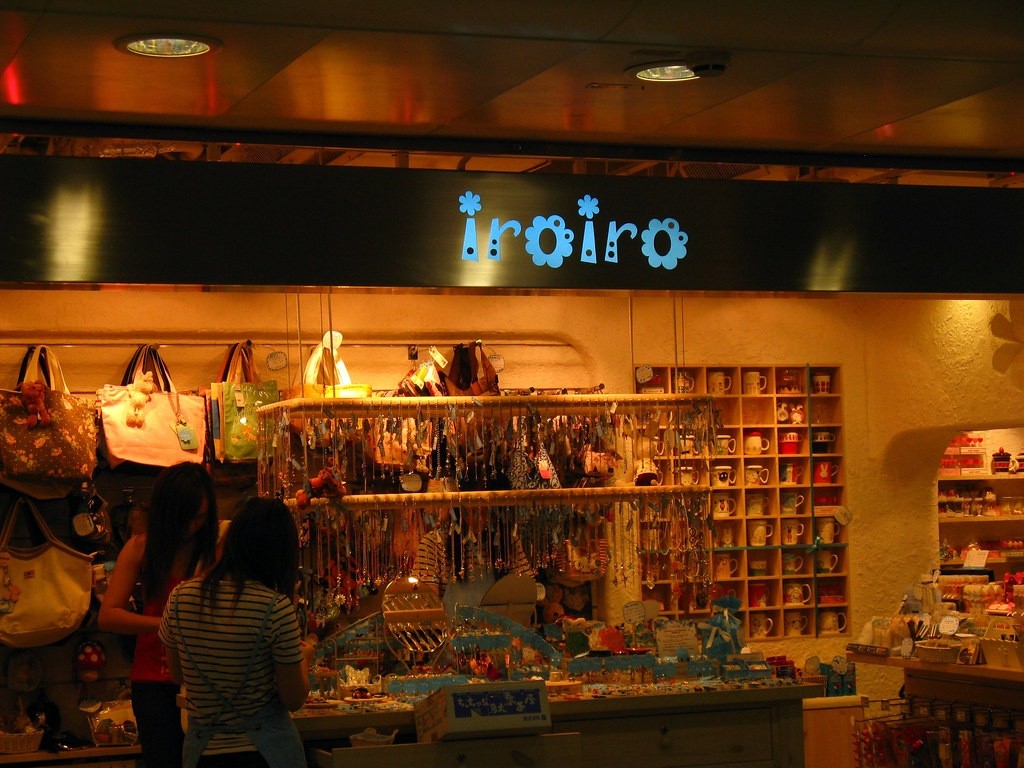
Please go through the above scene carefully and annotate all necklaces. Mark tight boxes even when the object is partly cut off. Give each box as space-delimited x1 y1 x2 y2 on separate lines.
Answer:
258 401 717 631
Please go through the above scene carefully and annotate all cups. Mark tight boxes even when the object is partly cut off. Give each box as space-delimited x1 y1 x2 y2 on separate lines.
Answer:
635 371 848 637
350 727 394 747
1012 453 1024 474
992 447 1017 475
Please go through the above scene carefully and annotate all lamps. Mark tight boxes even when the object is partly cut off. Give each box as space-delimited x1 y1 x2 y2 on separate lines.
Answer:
113 33 225 59
623 60 700 82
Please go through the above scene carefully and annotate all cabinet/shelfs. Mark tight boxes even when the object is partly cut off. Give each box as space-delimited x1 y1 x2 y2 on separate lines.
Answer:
631 362 851 641
0 743 142 768
939 471 1024 573
802 696 869 768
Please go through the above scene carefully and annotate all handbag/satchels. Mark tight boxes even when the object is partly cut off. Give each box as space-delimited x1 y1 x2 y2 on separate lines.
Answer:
96 344 206 470
198 342 283 464
366 343 503 470
508 415 562 490
546 512 608 588
0 496 94 648
0 345 97 500
285 341 373 447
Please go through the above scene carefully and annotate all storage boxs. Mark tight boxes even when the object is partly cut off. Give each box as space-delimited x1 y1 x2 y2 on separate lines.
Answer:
938 447 992 475
415 679 552 744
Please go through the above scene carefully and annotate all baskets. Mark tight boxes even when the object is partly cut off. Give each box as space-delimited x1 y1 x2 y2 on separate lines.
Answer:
0 728 44 754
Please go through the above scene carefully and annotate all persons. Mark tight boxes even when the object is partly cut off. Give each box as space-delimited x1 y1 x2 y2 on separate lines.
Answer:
411 464 536 598
98 462 219 768
159 498 310 768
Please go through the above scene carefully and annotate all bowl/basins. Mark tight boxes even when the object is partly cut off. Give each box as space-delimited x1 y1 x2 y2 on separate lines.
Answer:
915 639 962 664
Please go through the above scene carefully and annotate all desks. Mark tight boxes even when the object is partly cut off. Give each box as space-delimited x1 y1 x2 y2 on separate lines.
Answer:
844 652 1024 712
179 680 822 768
